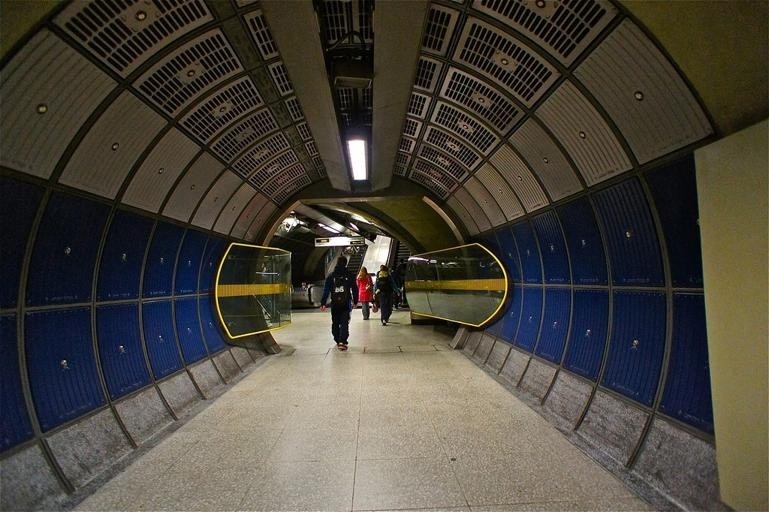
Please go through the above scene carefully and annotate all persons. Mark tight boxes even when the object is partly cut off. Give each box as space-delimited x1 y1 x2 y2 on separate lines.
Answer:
320 256 359 349
356 259 407 326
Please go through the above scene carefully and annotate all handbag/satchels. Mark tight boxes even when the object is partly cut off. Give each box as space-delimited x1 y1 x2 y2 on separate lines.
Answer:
366 283 373 293
372 302 378 312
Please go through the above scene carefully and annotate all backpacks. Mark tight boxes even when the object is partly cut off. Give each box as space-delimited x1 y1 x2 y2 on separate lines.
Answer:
331 272 351 305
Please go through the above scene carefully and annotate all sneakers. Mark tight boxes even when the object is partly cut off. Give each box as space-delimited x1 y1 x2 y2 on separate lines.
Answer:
382 320 386 325
338 342 347 351
